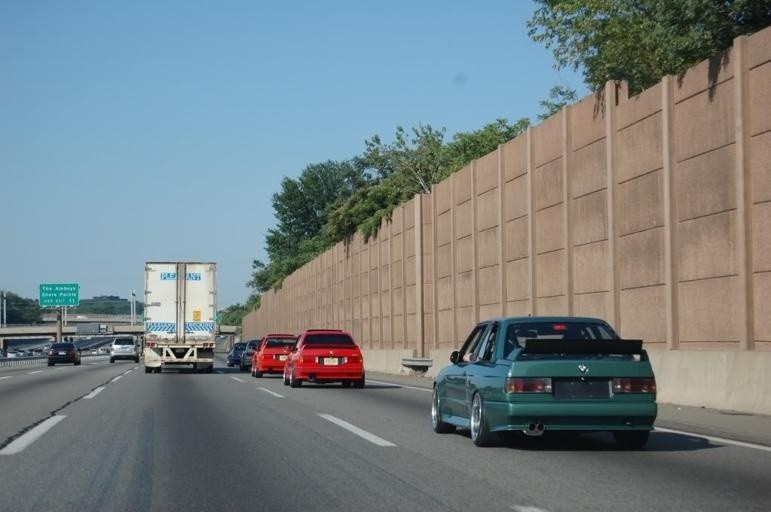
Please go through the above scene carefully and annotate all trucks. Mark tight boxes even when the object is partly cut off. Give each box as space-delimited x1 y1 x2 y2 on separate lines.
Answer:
144 262 216 374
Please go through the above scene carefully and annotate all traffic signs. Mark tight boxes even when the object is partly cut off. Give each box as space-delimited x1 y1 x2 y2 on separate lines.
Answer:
40 284 79 307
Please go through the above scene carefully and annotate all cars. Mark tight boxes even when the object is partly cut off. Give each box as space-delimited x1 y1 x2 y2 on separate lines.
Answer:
6 345 49 358
228 330 365 389
431 316 658 449
48 343 82 366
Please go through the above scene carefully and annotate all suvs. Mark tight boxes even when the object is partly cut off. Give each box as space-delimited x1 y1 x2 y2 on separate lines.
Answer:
109 336 140 363
